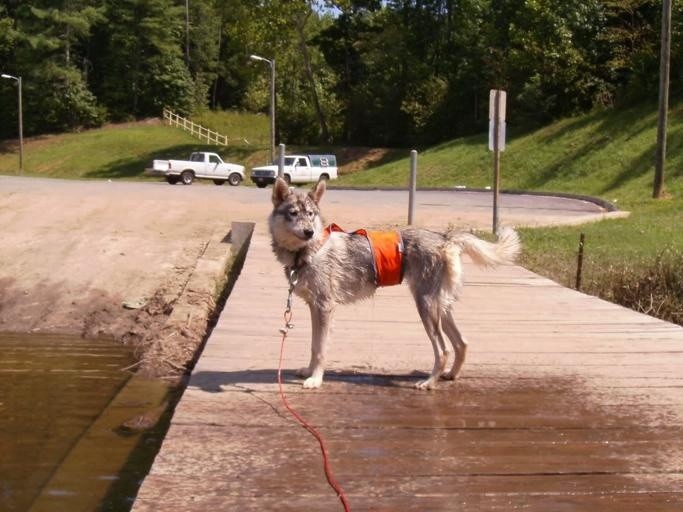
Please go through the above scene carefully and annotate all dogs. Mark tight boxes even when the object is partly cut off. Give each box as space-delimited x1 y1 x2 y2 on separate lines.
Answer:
266 174 522 393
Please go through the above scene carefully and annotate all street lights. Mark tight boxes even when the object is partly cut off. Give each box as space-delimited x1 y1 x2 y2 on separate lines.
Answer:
249 53 275 163
1 74 22 166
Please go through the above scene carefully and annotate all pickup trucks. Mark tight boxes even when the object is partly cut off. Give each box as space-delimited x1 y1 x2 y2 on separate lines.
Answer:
154 152 246 185
250 154 338 188
